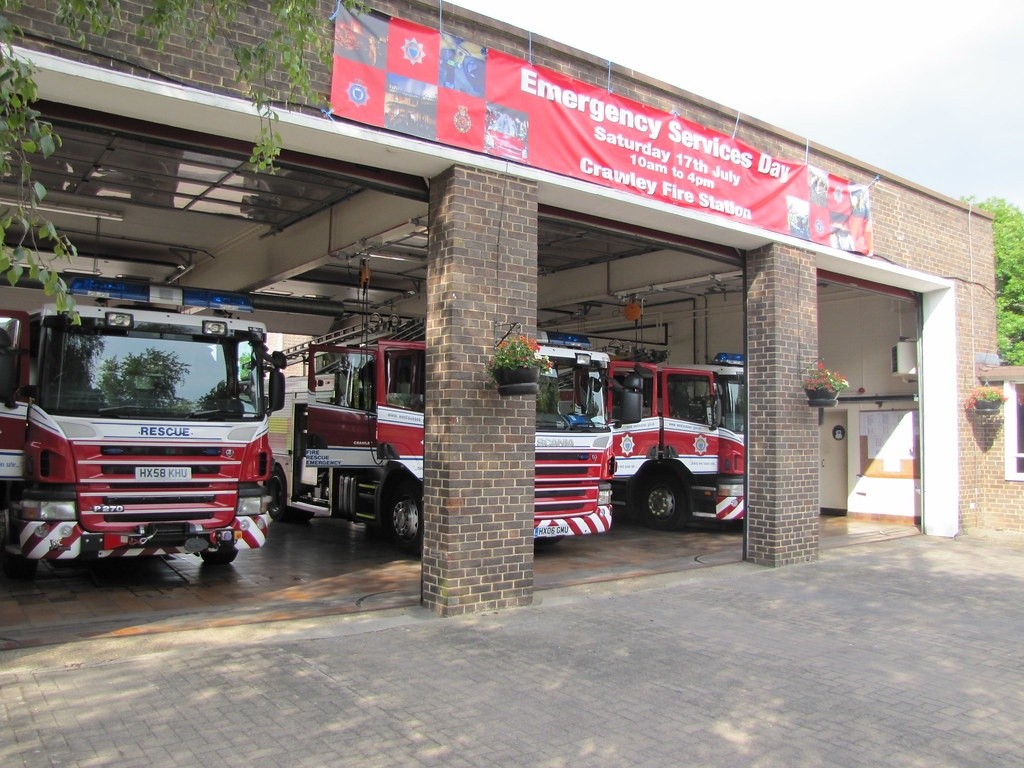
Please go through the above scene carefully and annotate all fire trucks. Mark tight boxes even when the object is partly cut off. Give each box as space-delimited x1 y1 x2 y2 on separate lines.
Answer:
598 338 746 522
261 312 644 558
1 275 288 576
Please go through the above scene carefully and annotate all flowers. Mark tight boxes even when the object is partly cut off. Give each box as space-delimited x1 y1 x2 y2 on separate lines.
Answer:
804 363 850 393
965 386 1008 408
487 334 551 373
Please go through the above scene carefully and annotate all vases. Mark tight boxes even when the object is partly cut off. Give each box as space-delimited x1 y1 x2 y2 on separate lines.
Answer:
806 389 839 408
497 366 539 395
975 400 1000 414
